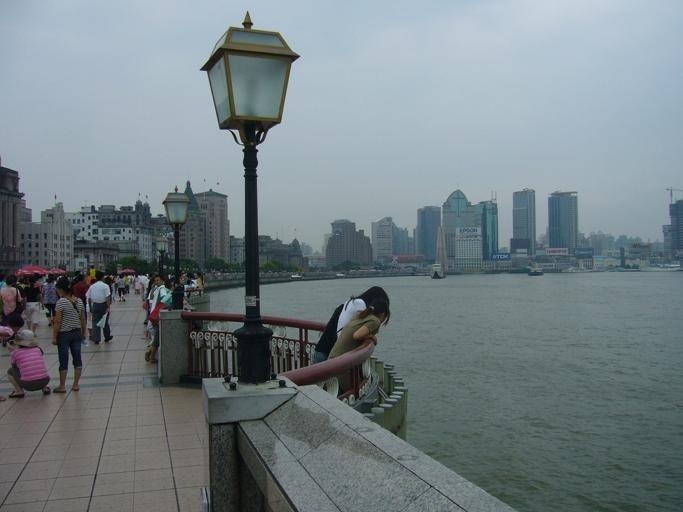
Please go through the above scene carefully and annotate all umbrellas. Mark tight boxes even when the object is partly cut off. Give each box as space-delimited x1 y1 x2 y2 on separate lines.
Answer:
13 263 64 277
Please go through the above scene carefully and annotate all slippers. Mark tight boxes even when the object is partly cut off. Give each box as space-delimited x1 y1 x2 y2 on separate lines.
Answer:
72 387 79 391
52 387 67 393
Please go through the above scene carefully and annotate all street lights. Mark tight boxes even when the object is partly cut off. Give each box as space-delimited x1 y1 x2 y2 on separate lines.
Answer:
157 187 192 310
201 11 299 380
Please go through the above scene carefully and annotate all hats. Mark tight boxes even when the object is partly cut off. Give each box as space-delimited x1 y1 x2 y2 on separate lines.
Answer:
14 329 39 347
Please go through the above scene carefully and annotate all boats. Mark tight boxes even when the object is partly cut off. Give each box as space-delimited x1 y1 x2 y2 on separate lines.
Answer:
430 263 445 278
526 262 543 275
560 266 588 273
610 267 640 273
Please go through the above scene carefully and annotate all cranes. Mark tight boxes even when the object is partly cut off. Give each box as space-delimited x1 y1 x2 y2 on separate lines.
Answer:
662 184 683 204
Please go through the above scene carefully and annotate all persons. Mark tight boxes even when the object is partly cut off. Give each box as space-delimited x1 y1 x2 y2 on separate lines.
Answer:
7 330 51 398
51 276 87 393
0 265 205 363
310 286 389 396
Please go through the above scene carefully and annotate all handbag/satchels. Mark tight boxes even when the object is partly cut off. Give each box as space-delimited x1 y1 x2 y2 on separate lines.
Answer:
15 288 27 314
146 302 168 321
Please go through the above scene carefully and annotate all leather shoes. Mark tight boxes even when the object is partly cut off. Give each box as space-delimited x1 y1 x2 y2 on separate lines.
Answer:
8 390 25 398
94 336 113 345
42 386 51 395
